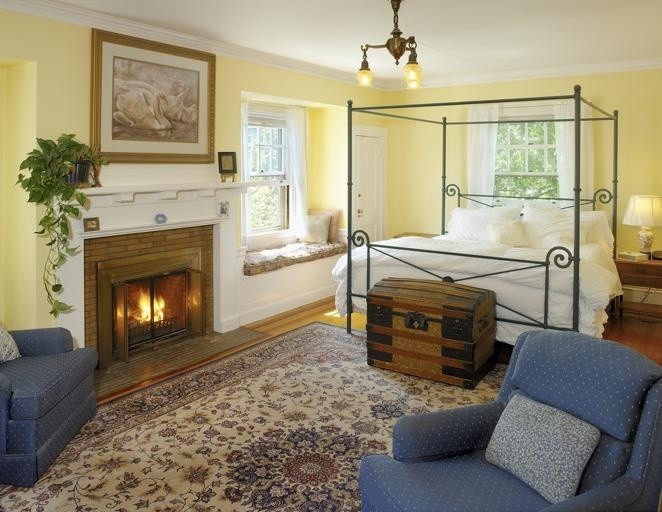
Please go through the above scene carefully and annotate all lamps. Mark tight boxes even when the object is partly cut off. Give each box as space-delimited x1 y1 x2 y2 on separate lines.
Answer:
623 195 662 259
358 0 422 89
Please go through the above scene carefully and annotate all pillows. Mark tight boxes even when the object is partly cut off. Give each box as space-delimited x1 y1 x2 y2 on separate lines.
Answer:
299 215 331 245
0 325 22 364
446 202 613 248
485 395 601 504
311 208 340 243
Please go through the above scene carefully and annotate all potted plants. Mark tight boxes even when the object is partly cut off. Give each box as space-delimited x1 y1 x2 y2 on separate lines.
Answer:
15 135 108 317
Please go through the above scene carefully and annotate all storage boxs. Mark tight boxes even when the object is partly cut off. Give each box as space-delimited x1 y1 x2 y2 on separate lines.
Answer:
366 277 497 389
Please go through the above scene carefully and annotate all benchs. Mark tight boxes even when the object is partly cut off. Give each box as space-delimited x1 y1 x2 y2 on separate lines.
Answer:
244 238 349 325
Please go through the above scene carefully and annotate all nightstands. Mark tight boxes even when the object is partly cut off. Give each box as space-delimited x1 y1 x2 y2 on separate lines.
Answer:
613 259 662 318
393 233 440 238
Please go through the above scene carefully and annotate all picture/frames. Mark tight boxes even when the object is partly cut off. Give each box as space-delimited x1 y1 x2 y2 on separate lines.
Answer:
218 152 237 174
91 28 216 164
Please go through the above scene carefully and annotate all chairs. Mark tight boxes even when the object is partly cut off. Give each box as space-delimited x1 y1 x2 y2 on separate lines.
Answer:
0 327 98 488
359 330 662 512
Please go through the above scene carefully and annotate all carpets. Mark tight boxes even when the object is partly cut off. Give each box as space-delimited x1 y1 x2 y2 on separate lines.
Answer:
0 322 509 512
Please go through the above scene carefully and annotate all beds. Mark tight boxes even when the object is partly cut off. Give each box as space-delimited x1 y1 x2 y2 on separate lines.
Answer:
332 86 623 348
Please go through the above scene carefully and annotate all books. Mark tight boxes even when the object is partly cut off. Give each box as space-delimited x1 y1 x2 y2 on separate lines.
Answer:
616 251 649 262
